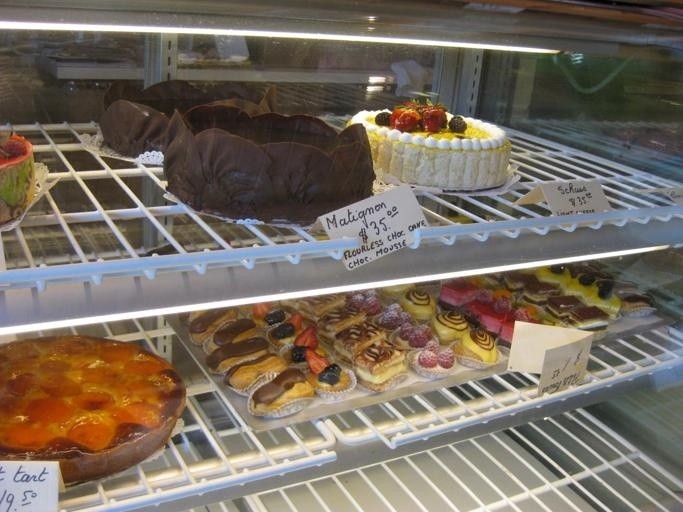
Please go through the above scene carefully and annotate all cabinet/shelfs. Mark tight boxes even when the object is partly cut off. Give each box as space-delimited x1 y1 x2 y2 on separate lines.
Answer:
0 0 682 512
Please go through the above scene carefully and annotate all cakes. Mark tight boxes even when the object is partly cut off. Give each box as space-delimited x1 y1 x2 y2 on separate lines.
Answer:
0 134 35 223
101 80 277 158
345 101 512 191
161 104 374 220
0 335 186 482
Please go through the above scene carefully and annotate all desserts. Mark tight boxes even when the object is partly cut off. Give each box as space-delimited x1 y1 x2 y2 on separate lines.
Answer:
179 264 656 418
